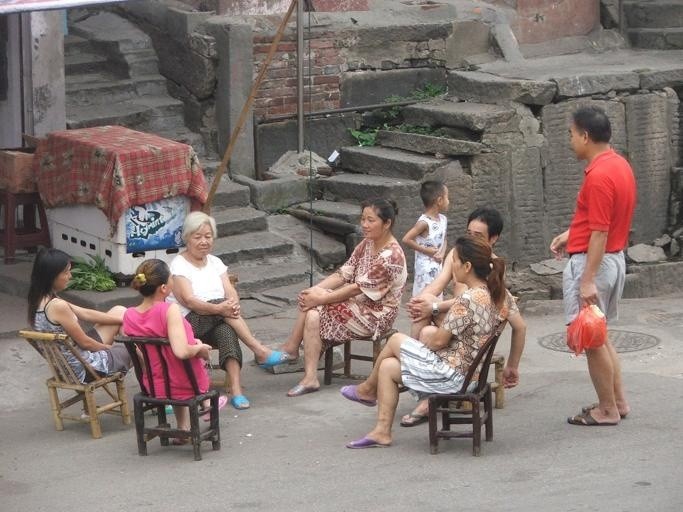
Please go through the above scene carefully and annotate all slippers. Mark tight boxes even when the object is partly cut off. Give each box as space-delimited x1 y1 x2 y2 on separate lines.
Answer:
231 395 249 409
259 350 289 368
340 384 377 406
287 384 320 397
567 409 618 425
346 436 388 449
400 412 428 427
582 403 597 412
271 346 297 364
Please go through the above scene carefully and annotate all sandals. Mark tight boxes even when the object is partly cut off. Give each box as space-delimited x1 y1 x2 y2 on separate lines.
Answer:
203 395 228 421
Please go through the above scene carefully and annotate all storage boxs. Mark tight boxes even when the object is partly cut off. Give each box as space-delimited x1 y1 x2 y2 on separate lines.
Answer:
0 133 41 192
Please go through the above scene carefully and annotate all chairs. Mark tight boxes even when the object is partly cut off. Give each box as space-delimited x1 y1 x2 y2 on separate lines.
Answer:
207 276 237 395
427 319 506 456
458 290 518 408
17 331 130 439
114 335 219 460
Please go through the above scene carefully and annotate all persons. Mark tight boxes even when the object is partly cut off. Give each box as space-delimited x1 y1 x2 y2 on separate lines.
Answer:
165 211 298 411
277 196 408 398
401 180 450 301
550 107 637 427
122 258 228 446
28 248 133 386
339 235 527 449
399 207 506 428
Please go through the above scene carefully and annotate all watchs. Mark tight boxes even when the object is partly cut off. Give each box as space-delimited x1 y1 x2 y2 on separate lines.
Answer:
431 302 440 317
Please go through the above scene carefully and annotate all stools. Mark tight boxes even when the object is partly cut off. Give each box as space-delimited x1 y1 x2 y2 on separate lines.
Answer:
324 329 399 384
0 191 53 263
